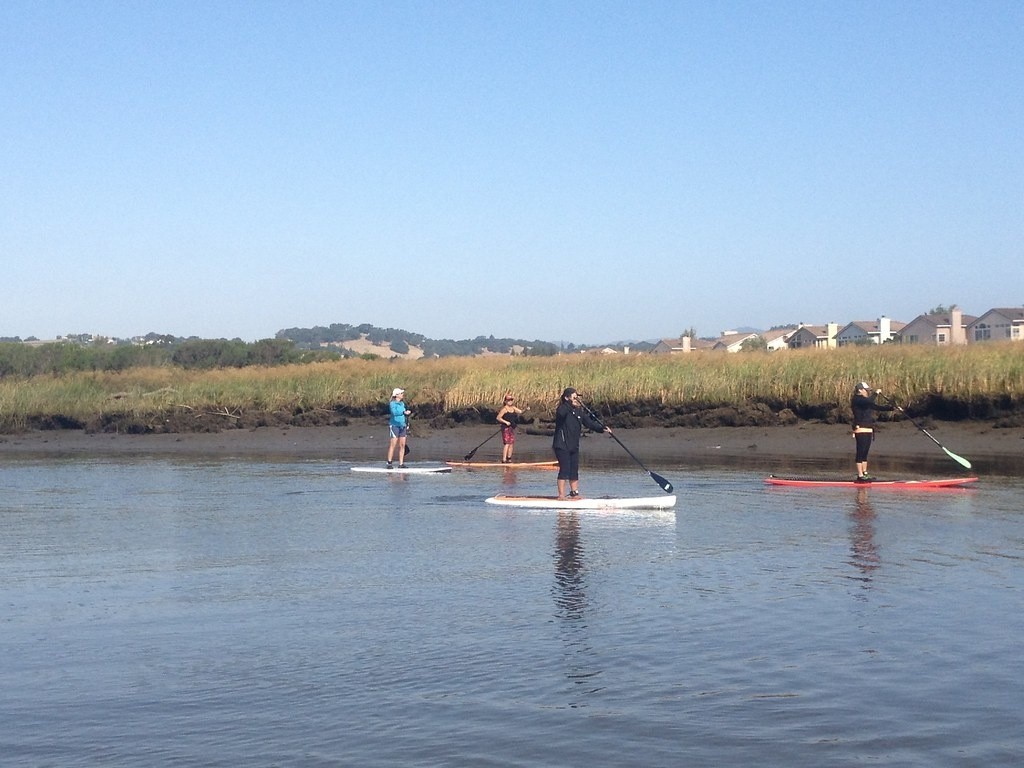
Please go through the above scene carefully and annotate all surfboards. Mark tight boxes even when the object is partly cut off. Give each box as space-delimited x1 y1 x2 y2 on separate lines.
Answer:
446 460 558 467
350 466 452 473
485 493 676 509
765 475 980 487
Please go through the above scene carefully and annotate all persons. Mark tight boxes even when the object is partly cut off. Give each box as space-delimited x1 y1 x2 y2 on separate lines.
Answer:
850 381 905 481
552 388 613 501
386 385 411 469
496 394 531 464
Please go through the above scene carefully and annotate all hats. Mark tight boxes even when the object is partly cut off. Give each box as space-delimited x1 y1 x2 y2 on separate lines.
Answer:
856 382 873 391
392 387 405 397
563 388 583 396
505 395 514 400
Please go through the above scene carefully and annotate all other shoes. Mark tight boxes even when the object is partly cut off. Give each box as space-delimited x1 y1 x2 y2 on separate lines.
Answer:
398 464 409 468
857 471 876 481
386 461 394 469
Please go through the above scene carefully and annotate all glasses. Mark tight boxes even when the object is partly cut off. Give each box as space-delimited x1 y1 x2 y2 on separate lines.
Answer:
398 392 404 395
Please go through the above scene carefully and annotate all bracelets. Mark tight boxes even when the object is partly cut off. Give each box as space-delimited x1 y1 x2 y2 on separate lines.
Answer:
403 410 405 414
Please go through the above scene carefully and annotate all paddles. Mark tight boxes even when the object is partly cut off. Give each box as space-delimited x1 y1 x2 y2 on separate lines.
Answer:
575 396 674 493
464 409 527 461
403 409 410 456
880 391 972 470
